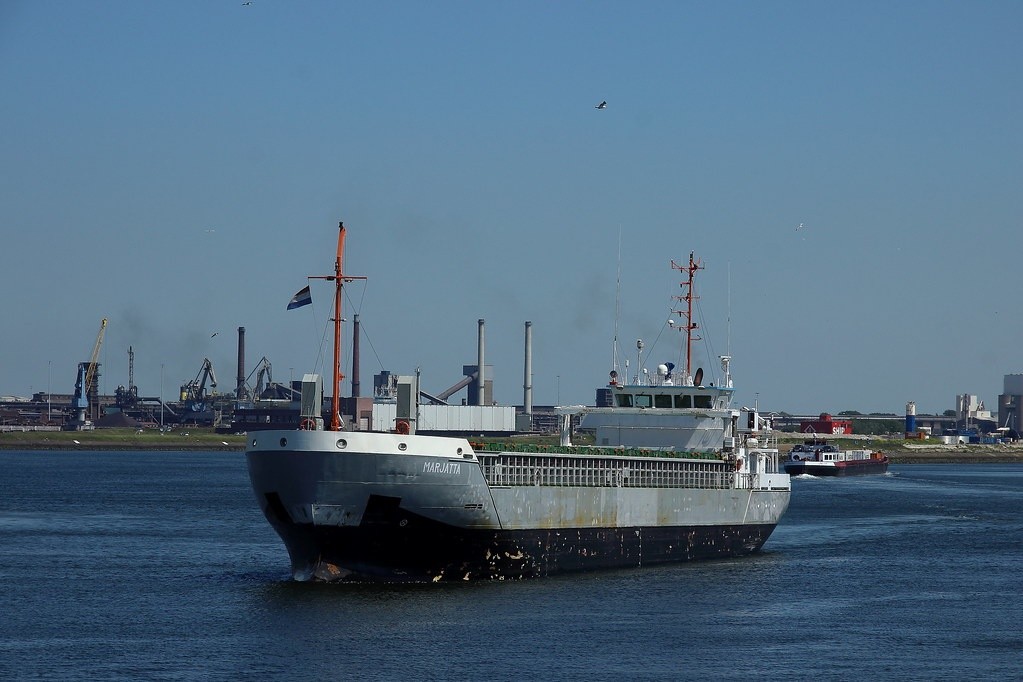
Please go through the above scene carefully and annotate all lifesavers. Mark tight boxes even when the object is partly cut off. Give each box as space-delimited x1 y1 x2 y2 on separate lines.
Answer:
300 418 315 430
396 421 410 433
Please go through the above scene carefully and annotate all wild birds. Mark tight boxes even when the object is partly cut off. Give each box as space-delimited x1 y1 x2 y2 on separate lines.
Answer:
594 100 608 109
211 333 218 338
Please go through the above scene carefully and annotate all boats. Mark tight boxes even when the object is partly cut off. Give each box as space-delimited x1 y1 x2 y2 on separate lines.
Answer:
245 221 792 580
784 432 891 477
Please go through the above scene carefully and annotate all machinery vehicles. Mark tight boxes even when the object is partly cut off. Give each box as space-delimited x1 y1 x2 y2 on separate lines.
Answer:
72 318 107 430
184 357 217 413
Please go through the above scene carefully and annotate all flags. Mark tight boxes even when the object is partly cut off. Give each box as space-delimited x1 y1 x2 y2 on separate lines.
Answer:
287 285 312 311
